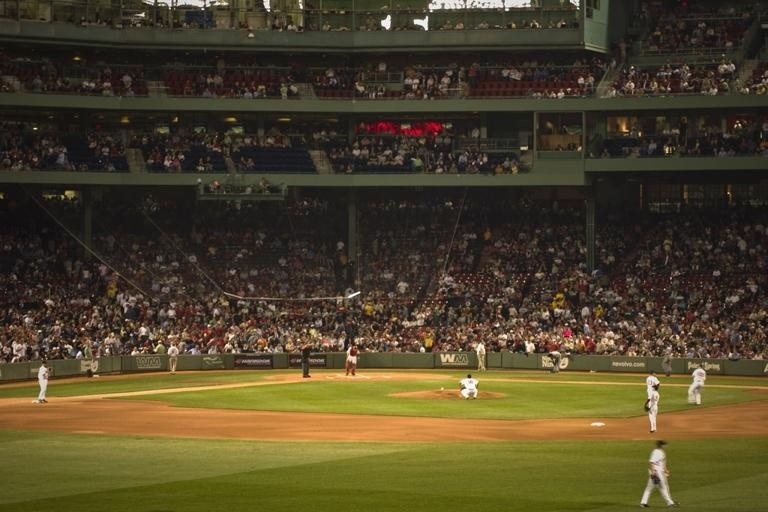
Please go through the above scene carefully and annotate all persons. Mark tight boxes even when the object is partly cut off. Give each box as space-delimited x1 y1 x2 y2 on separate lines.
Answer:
37 360 50 403
687 363 705 405
2 18 768 376
168 343 179 375
476 341 488 372
646 370 659 399
458 374 480 399
638 438 680 508
649 386 660 432
546 351 562 375
301 343 311 378
345 344 359 375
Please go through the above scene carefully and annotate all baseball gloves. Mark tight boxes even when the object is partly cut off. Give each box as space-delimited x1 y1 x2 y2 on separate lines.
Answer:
651 473 661 485
